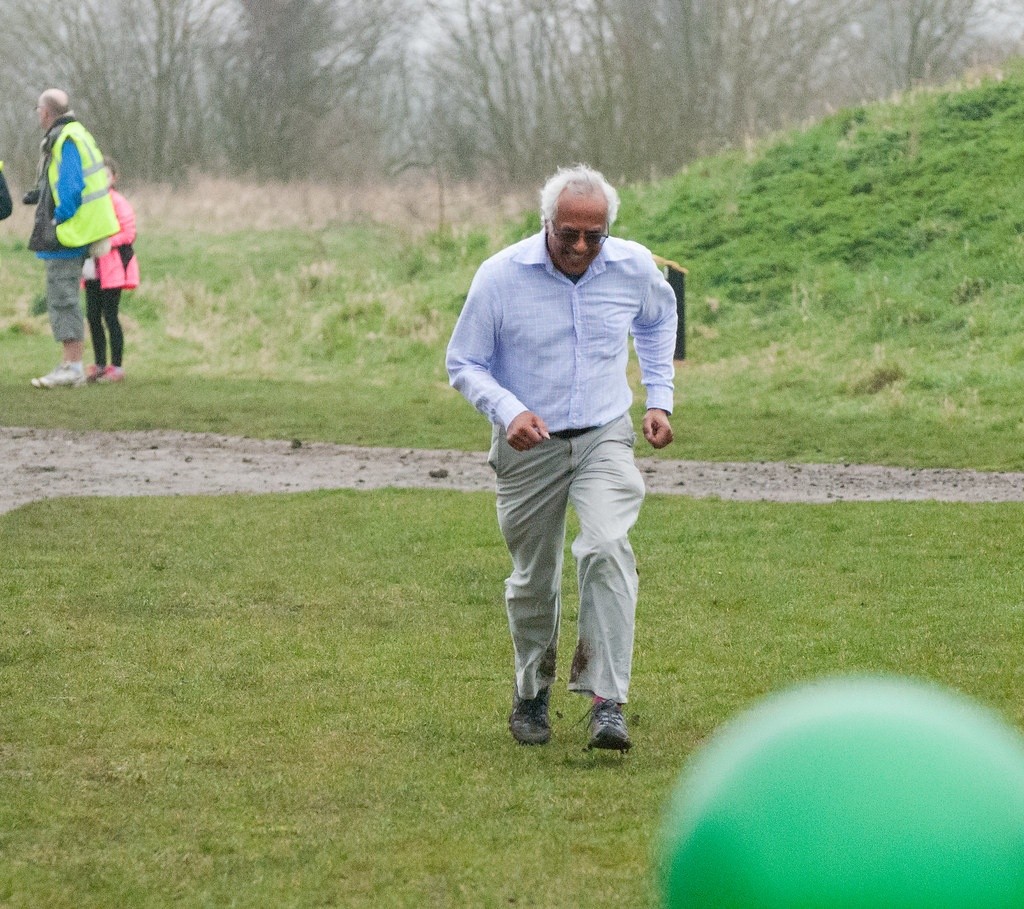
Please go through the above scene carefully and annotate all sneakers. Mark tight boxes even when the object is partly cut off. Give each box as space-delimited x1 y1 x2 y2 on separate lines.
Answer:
510 680 551 745
570 699 634 749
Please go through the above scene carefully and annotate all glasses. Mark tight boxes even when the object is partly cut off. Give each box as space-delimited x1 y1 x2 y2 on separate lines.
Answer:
552 219 610 246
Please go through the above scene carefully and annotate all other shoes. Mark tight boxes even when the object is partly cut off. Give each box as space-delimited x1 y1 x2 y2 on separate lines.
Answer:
85 365 105 379
30 362 87 388
96 366 123 384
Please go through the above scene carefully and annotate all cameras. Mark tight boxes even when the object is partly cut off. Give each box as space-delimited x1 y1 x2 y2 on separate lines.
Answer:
22 188 40 205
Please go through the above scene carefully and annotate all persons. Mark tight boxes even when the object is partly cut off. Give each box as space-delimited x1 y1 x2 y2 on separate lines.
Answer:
447 164 678 747
0 161 12 220
24 89 119 387
79 157 139 383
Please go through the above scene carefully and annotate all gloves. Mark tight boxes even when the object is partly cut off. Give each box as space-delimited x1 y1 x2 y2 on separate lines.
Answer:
87 239 110 258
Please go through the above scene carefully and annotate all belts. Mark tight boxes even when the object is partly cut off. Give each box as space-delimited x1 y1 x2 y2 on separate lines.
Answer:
553 425 600 439
